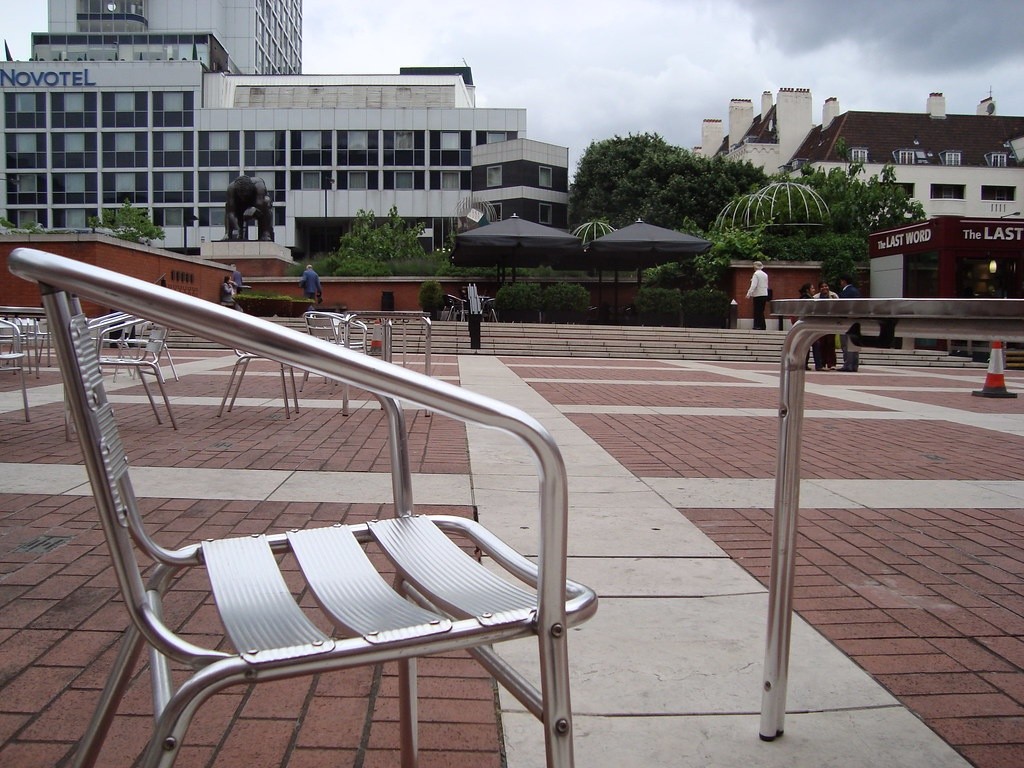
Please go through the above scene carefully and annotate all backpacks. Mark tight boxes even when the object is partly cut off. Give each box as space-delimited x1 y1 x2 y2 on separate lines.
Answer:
791 317 798 324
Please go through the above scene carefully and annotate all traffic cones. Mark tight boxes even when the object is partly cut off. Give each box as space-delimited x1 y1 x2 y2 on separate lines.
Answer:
367 318 383 356
971 341 1018 399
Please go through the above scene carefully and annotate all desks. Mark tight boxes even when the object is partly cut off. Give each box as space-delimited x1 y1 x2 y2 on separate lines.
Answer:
342 311 433 417
759 300 1024 743
0 305 46 379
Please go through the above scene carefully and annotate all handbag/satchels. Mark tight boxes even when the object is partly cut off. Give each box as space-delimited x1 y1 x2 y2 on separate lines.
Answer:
768 289 772 301
835 334 841 349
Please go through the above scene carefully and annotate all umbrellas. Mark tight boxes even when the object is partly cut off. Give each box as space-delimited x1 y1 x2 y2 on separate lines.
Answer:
450 213 582 282
583 217 713 286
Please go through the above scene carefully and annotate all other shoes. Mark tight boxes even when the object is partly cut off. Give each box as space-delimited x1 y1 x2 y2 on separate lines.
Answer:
816 368 824 371
753 327 766 330
806 368 811 370
836 368 858 371
831 366 836 370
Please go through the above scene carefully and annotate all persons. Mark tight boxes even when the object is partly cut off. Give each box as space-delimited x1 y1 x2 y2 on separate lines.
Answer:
224 175 274 241
799 283 815 370
746 261 768 330
232 271 242 293
222 276 243 312
300 264 322 317
813 281 839 371
838 272 861 372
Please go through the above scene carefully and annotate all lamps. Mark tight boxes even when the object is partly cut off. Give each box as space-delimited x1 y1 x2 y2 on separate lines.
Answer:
989 260 996 274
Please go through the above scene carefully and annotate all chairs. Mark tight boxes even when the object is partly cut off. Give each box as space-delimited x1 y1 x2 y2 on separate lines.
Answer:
0 247 598 766
